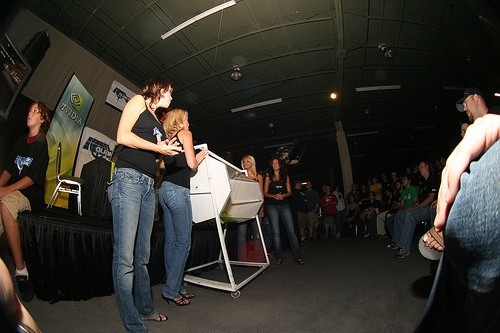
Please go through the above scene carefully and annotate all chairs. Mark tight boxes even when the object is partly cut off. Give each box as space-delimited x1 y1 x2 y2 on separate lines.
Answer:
315 207 430 240
47 142 84 217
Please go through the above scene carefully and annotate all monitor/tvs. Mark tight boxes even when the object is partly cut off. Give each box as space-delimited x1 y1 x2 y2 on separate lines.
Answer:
0 33 32 120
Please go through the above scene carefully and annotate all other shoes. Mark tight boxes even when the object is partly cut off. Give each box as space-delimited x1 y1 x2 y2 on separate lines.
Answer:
300 235 316 243
364 233 393 249
16 266 35 303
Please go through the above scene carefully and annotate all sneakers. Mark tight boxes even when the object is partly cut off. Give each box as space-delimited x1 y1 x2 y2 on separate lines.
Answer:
391 242 412 259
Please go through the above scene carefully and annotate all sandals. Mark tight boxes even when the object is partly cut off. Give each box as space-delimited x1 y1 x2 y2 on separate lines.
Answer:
161 290 195 306
275 257 283 265
152 313 169 322
418 226 445 261
294 257 305 265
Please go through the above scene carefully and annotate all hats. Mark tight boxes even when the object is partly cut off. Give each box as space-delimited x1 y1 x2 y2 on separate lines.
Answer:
455 87 481 112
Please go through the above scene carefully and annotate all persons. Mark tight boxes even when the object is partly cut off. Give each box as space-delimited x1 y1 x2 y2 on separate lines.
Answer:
320 186 339 239
264 156 304 265
415 88 500 333
107 78 183 333
346 157 446 240
238 155 265 259
392 161 439 259
0 102 50 302
385 176 419 249
332 185 346 239
158 109 207 306
290 181 327 247
